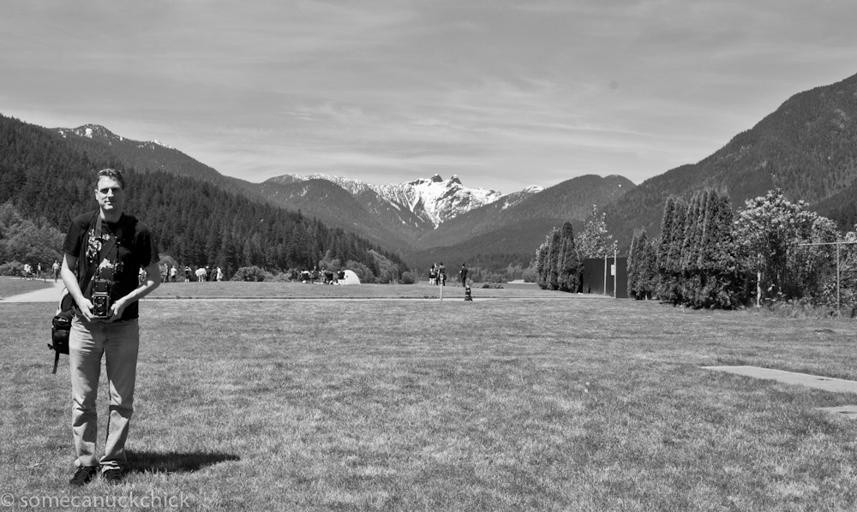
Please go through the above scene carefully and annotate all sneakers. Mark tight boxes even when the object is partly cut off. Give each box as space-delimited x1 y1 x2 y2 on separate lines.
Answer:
104 469 122 484
70 466 96 486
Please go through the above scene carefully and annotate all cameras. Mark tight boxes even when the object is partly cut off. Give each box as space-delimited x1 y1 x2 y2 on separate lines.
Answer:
91 293 110 315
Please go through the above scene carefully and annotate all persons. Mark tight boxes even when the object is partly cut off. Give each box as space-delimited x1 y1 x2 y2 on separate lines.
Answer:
138 262 223 282
60 168 162 486
23 260 60 283
288 265 345 285
428 263 446 285
459 264 468 286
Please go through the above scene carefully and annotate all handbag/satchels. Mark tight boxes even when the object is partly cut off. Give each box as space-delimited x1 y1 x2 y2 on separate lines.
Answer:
47 285 75 354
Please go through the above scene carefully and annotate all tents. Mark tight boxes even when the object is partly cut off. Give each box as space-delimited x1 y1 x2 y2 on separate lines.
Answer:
330 269 360 285
